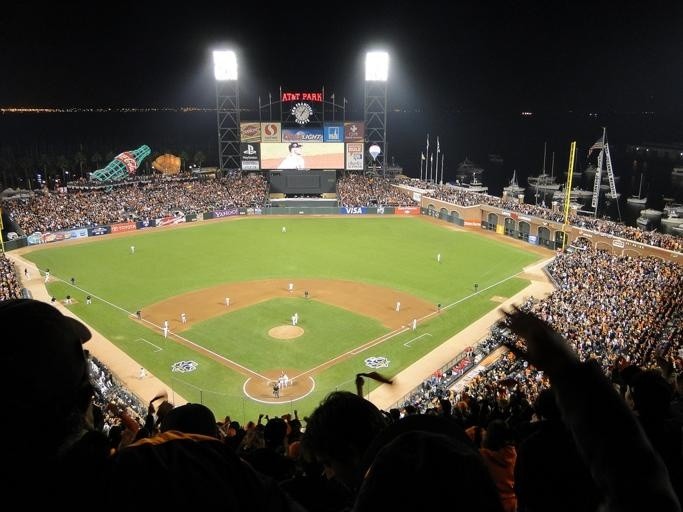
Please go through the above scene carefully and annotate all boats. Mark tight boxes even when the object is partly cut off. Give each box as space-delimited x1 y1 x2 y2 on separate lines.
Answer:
456 140 683 234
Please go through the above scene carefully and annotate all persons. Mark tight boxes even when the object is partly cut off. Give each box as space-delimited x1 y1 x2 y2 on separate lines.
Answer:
340 175 416 209
2 170 270 241
277 142 304 169
0 245 683 511
400 177 683 254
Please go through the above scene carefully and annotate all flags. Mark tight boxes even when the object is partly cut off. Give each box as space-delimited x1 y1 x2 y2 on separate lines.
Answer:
421 153 426 160
585 135 604 161
425 134 430 149
437 136 441 153
430 153 433 161
